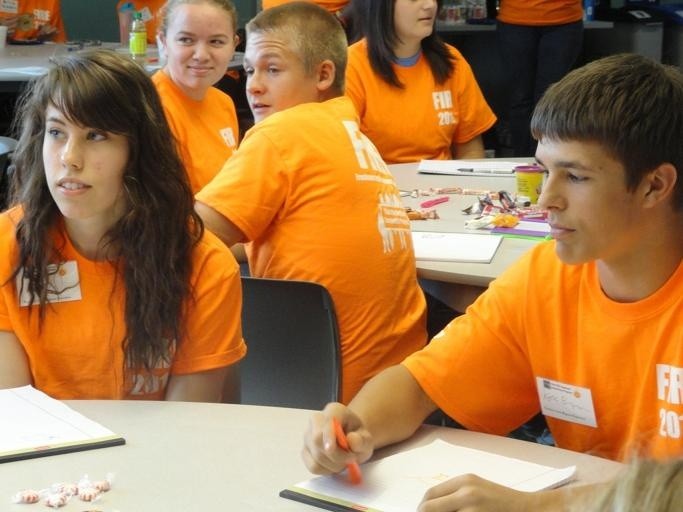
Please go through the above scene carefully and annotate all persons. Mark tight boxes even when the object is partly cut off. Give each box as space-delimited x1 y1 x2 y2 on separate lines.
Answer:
303 51 683 511
342 0 500 160
496 1 587 158
0 0 67 44
117 1 168 46
262 1 353 29
0 49 248 407
187 1 429 408
141 0 241 205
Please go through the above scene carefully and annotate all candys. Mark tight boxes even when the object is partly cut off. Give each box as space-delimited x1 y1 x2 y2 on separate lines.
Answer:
16 478 110 507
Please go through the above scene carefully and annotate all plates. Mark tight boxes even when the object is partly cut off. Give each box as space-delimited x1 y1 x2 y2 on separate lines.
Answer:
7 41 42 45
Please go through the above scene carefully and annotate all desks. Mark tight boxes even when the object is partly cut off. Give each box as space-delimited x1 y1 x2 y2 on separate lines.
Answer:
386 154 541 312
434 19 614 81
0 42 245 78
0 390 636 512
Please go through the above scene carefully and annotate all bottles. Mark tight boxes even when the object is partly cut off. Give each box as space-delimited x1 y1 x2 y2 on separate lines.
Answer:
126 12 147 67
437 2 485 26
582 0 594 22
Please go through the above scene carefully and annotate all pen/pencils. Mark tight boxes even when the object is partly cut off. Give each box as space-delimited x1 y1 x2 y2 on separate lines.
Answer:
457 167 516 173
333 416 363 485
421 196 449 209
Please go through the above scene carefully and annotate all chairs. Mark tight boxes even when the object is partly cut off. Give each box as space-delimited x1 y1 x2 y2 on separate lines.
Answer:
226 274 341 415
0 137 28 213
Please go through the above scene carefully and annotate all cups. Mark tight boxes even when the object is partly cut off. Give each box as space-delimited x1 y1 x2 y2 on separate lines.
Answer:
0 26 7 48
515 166 544 202
